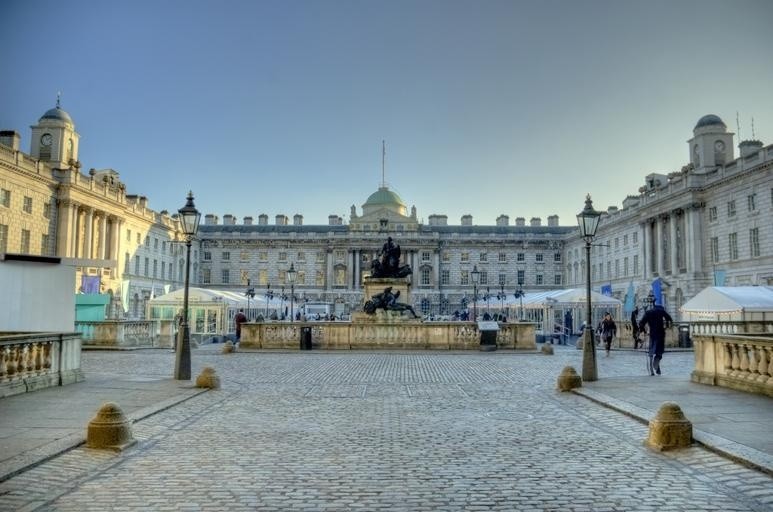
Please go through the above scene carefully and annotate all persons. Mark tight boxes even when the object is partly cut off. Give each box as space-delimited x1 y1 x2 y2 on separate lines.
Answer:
482 312 491 321
451 309 459 319
491 311 498 321
599 311 617 358
595 322 602 346
578 320 586 337
502 316 506 322
377 235 395 263
230 306 248 348
637 293 673 376
254 308 335 323
459 310 469 321
629 302 647 350
498 313 502 320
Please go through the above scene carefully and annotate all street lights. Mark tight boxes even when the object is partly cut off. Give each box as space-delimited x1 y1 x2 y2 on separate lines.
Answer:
461 264 526 322
575 192 601 381
244 261 309 322
173 190 201 381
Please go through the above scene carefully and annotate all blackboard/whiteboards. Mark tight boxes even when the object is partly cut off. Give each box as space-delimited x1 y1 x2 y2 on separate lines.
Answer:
480 330 496 345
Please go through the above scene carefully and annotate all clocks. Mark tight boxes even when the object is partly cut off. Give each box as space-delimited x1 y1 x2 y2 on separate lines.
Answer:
693 140 726 156
39 133 74 151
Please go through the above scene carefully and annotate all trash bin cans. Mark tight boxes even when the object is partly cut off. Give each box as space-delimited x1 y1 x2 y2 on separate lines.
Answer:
299 327 311 350
552 334 559 344
